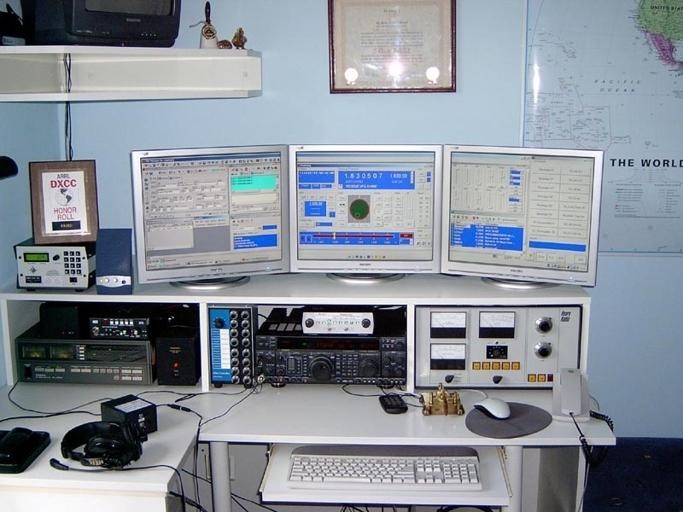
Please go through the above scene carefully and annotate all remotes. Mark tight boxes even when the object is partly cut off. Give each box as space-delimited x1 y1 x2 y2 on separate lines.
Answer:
379 392 408 414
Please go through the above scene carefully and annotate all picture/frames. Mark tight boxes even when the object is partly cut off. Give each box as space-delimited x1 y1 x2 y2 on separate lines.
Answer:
28 159 100 246
326 0 456 95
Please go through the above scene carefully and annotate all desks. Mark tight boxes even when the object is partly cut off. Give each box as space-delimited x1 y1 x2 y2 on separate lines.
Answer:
0 272 621 511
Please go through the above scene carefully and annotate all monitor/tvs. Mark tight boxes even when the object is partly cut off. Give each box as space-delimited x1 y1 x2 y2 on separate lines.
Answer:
20 1 181 48
441 145 604 290
288 144 442 285
132 145 288 290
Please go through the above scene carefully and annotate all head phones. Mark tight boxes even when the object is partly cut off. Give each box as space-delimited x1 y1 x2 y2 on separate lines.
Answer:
61 421 142 470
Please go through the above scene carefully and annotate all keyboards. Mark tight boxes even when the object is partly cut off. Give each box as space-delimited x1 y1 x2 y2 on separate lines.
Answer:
287 453 483 493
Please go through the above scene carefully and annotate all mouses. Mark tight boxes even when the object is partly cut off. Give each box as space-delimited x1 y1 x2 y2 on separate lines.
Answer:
475 397 511 420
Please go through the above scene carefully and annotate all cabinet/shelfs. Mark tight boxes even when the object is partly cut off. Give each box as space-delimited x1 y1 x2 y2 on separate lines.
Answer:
0 45 263 107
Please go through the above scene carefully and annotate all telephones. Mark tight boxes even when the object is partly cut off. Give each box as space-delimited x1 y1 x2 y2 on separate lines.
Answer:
550 367 591 422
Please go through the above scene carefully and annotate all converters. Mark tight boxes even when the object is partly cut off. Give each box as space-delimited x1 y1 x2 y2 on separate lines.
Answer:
100 394 157 436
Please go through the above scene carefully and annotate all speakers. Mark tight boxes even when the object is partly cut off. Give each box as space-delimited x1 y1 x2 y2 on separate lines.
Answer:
96 228 132 294
